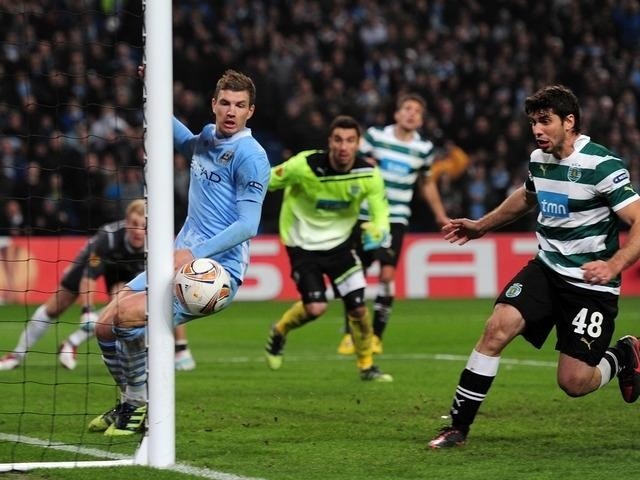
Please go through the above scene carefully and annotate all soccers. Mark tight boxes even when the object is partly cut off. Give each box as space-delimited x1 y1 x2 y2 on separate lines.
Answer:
175 257 230 316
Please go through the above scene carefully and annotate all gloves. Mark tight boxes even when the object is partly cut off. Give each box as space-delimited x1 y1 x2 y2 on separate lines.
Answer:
357 220 393 252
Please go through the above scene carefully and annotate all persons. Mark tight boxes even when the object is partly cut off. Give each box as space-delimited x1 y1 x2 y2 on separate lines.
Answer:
0 200 146 370
86 66 272 435
175 323 196 370
337 93 456 355
264 116 395 382
428 84 640 450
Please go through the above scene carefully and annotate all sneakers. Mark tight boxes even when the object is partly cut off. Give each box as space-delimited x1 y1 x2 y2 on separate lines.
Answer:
56 338 79 371
429 425 466 450
616 334 639 405
87 402 149 438
265 325 284 370
173 354 194 373
336 332 384 355
358 364 394 384
0 349 20 370
